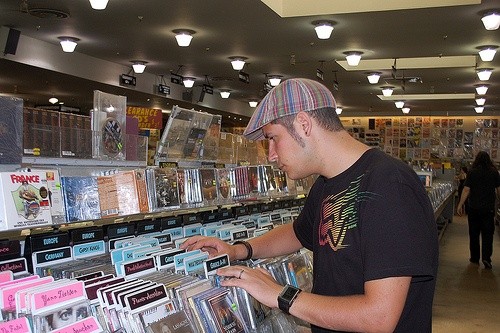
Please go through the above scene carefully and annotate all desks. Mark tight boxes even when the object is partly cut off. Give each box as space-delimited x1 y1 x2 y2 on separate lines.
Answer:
433 189 456 242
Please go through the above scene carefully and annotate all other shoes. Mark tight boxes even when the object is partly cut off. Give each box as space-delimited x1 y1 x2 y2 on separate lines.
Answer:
469 257 478 263
482 258 493 268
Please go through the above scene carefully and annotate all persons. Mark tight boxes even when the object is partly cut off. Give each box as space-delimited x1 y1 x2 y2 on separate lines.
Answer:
458 150 500 268
179 78 440 333
45 303 87 329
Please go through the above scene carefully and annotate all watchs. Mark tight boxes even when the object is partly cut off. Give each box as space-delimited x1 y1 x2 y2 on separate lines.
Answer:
277 284 302 315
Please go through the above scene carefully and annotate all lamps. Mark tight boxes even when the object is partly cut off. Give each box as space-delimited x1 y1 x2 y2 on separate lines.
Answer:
311 19 411 114
57 0 284 108
473 8 500 114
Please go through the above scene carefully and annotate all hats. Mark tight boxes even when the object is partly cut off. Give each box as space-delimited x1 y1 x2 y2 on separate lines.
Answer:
243 78 337 142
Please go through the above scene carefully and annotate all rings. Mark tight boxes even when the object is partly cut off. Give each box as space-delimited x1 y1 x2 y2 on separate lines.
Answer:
239 270 244 278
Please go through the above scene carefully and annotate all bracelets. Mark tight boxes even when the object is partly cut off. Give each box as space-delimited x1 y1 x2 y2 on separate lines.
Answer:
233 240 252 261
